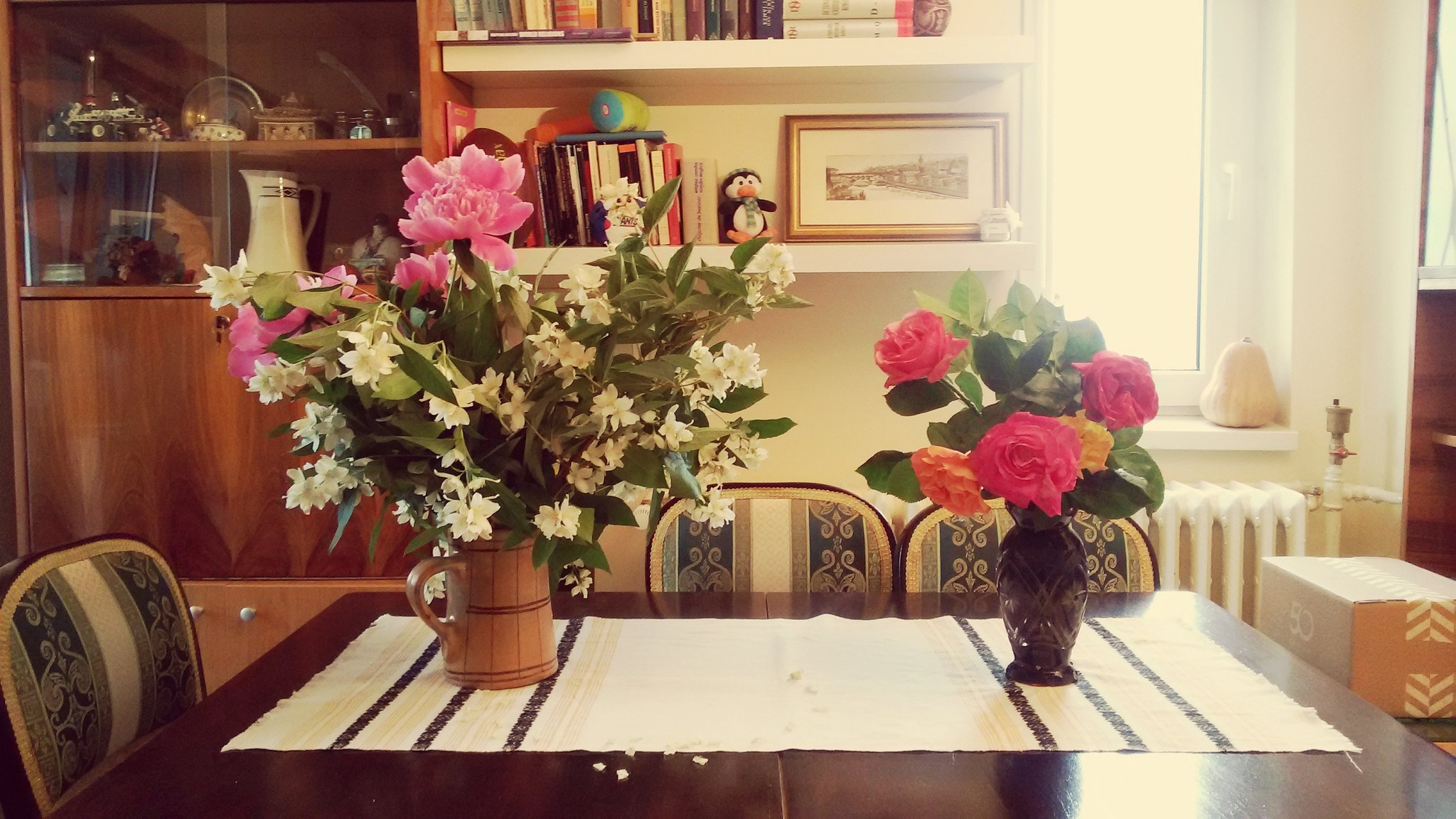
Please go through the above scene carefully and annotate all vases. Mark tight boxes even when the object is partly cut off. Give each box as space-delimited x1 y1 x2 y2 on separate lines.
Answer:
995 496 1090 687
405 522 558 690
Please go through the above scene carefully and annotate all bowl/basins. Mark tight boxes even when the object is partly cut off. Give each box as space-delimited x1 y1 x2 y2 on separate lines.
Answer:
188 123 247 141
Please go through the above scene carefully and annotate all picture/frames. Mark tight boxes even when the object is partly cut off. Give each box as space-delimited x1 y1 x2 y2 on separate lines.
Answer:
785 115 1007 244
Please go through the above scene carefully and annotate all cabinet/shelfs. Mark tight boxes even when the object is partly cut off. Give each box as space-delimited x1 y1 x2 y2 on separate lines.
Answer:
0 0 1026 698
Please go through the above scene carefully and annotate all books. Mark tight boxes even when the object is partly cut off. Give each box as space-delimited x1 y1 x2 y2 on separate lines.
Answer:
510 129 719 245
436 0 952 43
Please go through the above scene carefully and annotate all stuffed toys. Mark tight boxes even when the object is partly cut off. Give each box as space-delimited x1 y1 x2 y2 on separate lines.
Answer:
589 196 648 244
718 167 777 244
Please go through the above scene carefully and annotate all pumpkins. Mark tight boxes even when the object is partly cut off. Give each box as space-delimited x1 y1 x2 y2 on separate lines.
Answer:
1199 336 1285 427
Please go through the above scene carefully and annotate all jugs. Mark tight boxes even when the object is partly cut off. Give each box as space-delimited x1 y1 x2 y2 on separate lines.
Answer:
240 169 321 281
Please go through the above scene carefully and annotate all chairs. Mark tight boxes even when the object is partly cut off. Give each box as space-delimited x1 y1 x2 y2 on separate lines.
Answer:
645 483 897 592
893 489 1160 593
0 532 206 819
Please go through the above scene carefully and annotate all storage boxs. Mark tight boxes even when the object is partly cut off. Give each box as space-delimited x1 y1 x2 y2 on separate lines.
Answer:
1261 557 1456 719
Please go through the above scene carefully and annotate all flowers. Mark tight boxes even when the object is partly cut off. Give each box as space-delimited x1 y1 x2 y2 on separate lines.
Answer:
857 268 1164 519
194 144 814 600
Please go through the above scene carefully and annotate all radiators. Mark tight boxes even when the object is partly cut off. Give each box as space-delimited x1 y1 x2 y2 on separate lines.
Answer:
1131 398 1400 623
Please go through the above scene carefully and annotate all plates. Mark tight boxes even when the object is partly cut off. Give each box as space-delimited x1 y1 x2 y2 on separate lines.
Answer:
181 77 262 141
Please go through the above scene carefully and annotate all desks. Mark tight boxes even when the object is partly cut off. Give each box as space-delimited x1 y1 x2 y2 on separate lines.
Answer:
45 592 1456 819
